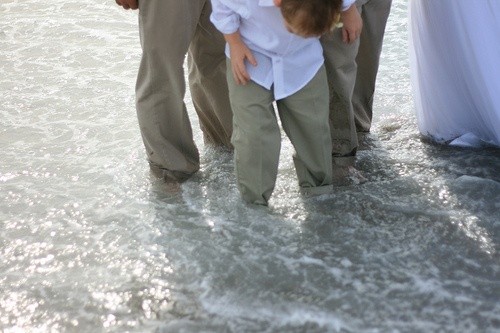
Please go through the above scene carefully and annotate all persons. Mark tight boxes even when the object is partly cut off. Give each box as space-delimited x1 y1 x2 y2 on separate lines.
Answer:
208 0 363 207
320 0 392 186
116 0 233 184
410 0 499 148
